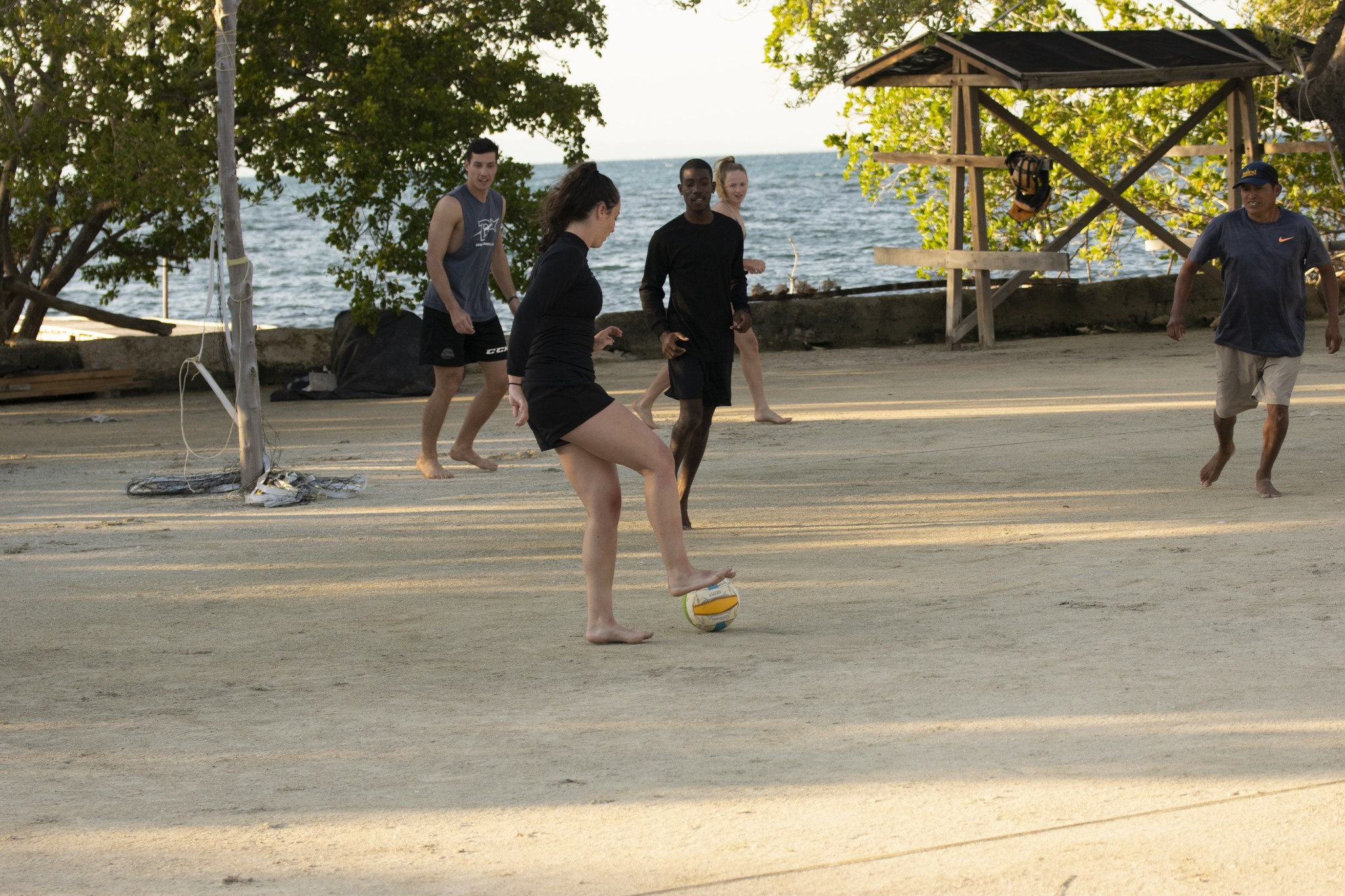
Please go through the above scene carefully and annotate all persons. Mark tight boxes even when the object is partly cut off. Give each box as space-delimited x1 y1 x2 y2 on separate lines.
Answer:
506 162 736 644
633 156 792 430
638 158 753 530
1166 161 1343 497
417 137 521 479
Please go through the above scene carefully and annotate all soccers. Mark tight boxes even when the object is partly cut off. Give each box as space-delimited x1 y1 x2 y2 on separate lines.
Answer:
682 581 741 631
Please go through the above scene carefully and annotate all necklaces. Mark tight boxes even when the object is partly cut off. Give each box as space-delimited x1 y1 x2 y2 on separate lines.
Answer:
730 203 740 209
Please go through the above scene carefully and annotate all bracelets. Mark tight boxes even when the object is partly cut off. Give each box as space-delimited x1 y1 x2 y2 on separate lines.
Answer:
508 383 522 386
507 296 518 304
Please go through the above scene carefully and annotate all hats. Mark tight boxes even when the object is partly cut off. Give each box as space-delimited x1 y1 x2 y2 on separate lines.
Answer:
1233 162 1278 189
